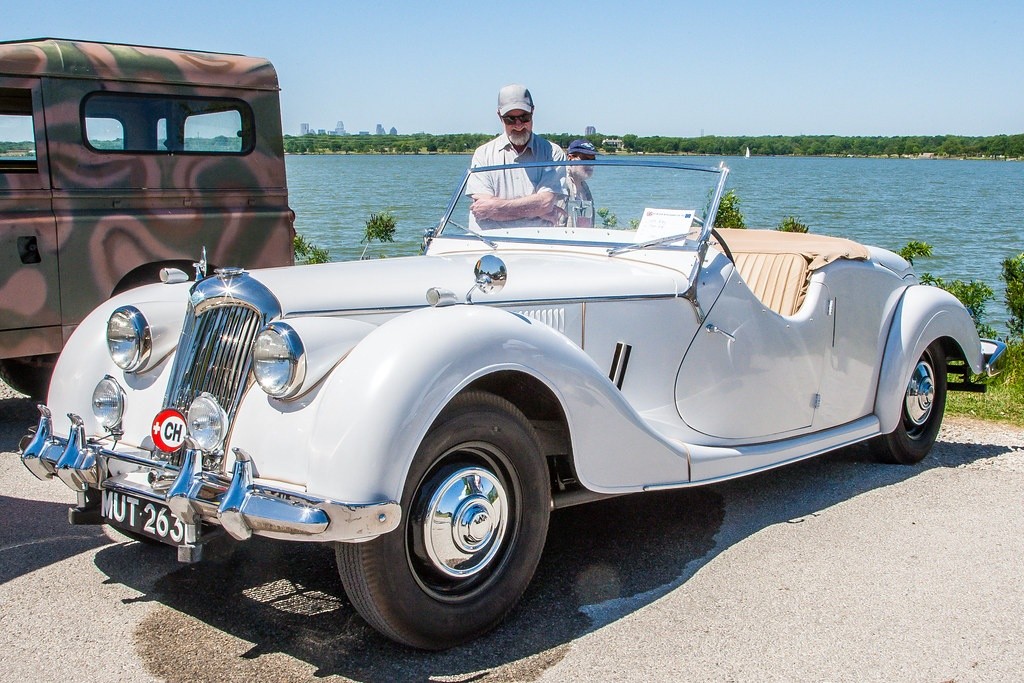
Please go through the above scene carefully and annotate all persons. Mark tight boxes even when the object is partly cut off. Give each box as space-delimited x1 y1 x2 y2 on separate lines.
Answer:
468 86 569 231
567 141 603 228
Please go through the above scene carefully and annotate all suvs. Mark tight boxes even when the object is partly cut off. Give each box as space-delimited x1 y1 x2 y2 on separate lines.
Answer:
0 36 296 395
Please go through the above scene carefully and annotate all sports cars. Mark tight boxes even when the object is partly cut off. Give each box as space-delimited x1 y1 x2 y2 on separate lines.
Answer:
22 162 1007 649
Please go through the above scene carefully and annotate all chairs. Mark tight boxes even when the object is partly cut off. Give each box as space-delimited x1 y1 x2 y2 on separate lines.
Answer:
718 252 808 319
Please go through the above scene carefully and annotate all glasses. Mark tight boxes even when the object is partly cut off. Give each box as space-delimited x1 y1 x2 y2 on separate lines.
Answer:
573 154 596 160
502 114 531 125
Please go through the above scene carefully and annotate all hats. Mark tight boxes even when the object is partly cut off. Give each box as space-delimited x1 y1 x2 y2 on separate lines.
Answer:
497 85 533 116
566 139 604 155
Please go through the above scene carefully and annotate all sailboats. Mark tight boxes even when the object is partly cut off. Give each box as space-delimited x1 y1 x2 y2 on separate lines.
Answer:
744 147 750 160
